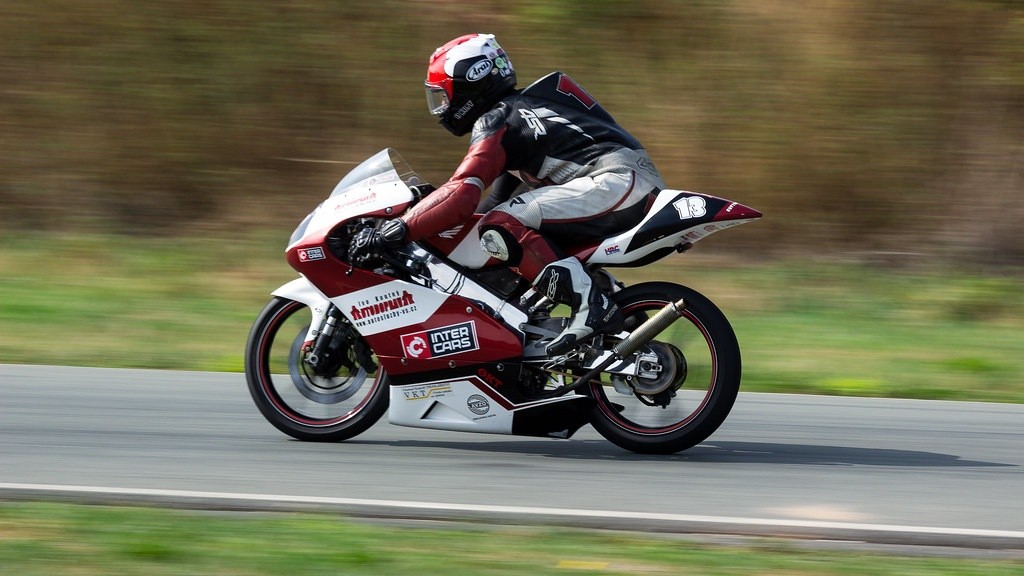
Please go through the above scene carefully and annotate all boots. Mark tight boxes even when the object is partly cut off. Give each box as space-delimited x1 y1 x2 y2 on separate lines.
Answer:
531 256 625 356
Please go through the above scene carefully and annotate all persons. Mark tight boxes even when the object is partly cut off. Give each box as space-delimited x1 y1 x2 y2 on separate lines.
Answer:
347 33 667 357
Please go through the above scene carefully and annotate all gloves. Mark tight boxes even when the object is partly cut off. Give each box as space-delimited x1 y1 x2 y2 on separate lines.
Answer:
348 217 408 269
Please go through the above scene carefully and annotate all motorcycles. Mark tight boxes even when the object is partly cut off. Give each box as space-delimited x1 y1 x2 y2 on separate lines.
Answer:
243 147 764 458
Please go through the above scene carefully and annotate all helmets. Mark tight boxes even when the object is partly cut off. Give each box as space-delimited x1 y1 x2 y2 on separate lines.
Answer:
424 34 517 136
